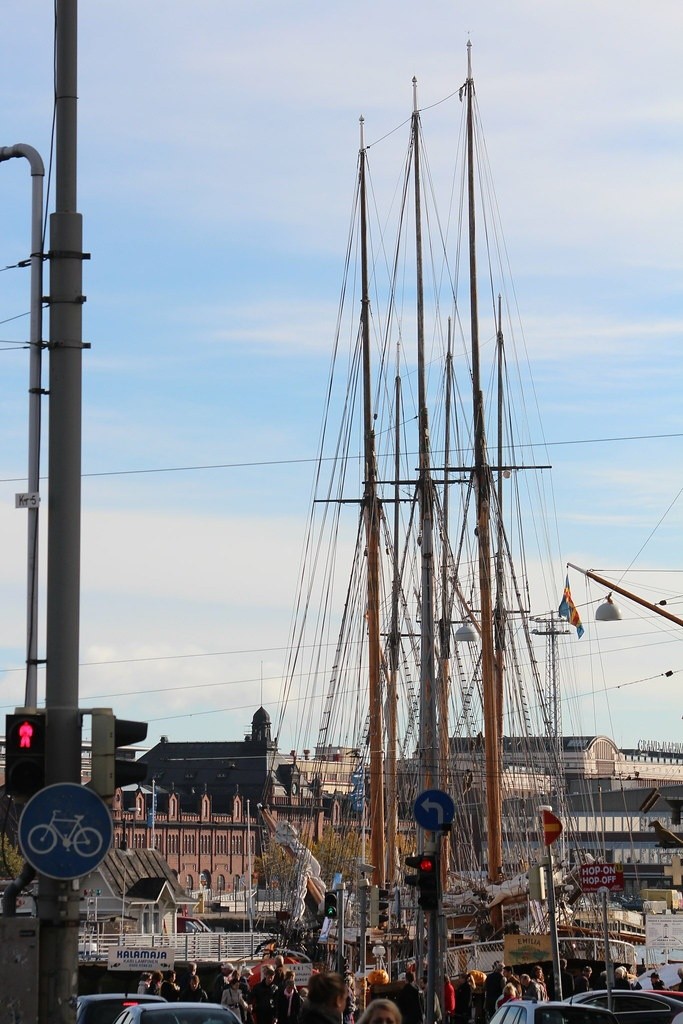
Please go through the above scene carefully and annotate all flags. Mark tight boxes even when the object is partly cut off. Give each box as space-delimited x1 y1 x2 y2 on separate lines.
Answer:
560 573 585 640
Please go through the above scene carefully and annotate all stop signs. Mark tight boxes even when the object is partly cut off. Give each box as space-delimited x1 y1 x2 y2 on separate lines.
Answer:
543 809 562 846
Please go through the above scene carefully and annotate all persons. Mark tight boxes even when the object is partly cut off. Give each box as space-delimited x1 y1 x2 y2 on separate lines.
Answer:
102 914 683 1024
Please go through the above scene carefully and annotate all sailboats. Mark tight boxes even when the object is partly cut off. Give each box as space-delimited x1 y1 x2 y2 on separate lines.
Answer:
256 39 683 989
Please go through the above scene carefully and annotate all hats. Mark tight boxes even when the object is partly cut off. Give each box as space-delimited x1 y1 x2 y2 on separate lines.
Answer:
223 963 235 970
647 972 659 978
243 968 254 976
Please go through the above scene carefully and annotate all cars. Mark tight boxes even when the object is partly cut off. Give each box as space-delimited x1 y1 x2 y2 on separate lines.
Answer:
77 994 168 1024
112 1002 241 1024
563 990 683 1024
488 996 619 1024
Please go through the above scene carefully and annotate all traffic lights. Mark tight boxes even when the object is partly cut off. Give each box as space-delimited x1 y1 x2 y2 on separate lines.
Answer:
379 889 388 923
114 715 148 789
325 892 338 917
5 714 48 799
405 854 422 887
418 855 438 911
